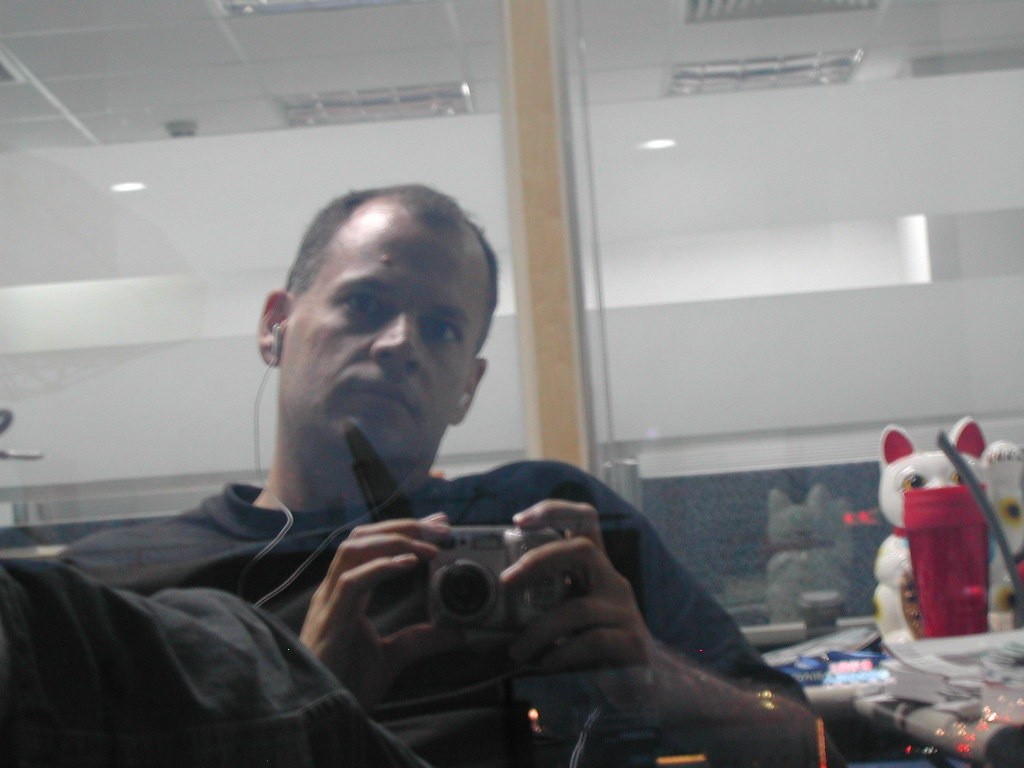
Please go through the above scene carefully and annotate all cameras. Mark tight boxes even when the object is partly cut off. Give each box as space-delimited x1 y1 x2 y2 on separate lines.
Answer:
425 522 569 629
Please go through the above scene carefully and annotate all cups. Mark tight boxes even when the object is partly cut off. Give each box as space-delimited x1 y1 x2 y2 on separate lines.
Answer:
903 485 990 638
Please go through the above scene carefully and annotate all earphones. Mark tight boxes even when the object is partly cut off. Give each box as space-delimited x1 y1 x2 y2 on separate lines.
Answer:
272 324 283 363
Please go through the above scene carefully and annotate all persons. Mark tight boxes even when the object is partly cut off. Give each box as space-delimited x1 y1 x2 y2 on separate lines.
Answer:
0 185 832 768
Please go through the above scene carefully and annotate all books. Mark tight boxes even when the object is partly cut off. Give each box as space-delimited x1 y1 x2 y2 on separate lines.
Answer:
882 626 1024 730
